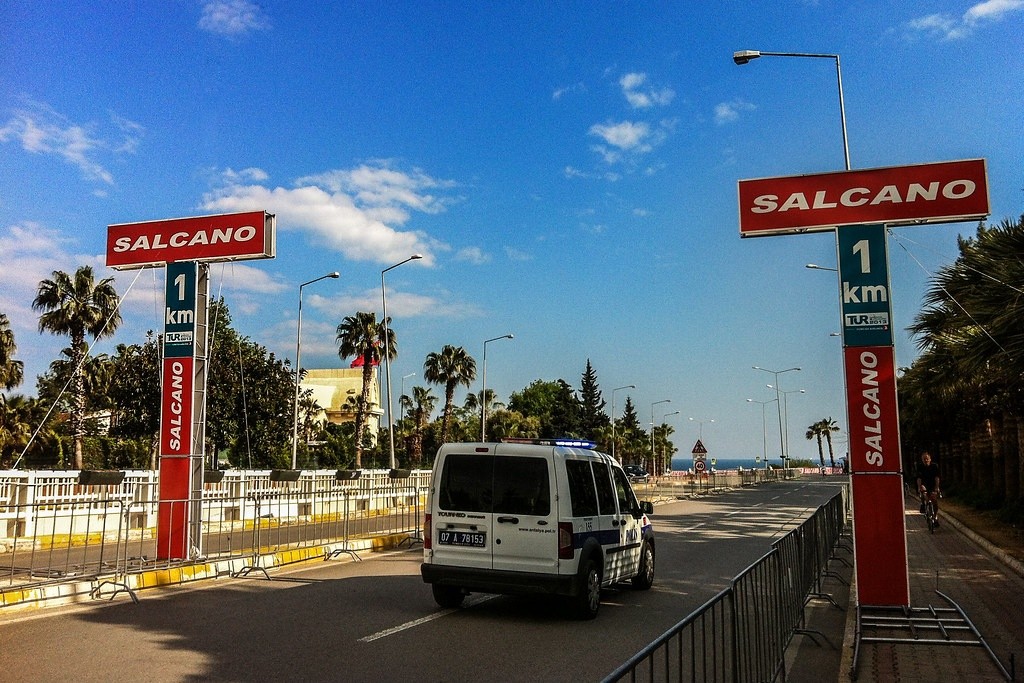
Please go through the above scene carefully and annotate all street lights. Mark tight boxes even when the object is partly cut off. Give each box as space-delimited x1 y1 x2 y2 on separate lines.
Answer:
749 366 802 471
612 384 636 457
399 371 415 450
479 333 515 443
688 417 716 442
766 384 805 468
746 398 778 469
651 399 671 477
291 270 340 471
664 411 680 474
732 49 853 170
381 253 423 470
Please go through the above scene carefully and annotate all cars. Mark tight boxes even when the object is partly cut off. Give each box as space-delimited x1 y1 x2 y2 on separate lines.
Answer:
623 464 650 483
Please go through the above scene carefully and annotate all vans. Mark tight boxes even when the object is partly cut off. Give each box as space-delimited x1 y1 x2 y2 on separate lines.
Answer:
420 438 656 621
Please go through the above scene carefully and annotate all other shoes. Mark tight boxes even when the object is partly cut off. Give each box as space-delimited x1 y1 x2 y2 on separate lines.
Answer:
934 522 940 527
920 503 926 512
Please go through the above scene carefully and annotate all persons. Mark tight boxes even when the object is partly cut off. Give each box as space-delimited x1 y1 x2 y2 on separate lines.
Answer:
917 451 942 529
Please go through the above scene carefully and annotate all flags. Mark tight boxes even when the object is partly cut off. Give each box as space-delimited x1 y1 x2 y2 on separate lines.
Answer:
350 343 380 369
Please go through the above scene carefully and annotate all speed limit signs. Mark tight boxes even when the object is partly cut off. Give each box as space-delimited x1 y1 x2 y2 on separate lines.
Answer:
694 460 706 472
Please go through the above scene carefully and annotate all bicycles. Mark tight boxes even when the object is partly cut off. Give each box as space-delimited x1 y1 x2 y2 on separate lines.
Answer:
919 489 942 534
